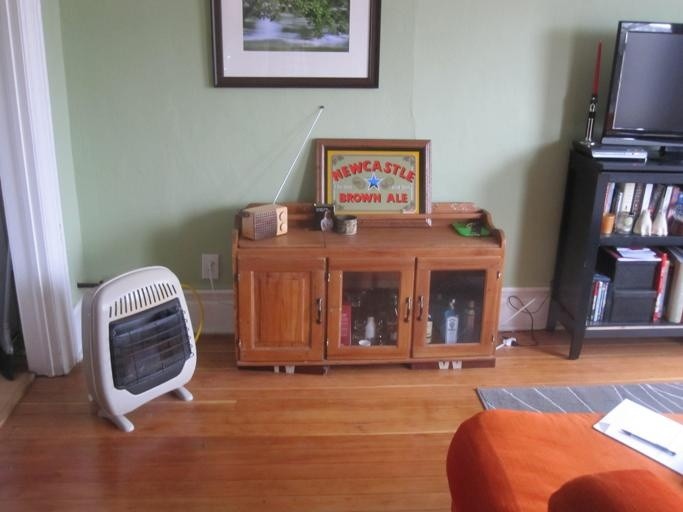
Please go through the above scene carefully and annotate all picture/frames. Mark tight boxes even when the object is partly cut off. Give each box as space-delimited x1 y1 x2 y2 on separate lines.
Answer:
210 1 381 91
312 137 433 226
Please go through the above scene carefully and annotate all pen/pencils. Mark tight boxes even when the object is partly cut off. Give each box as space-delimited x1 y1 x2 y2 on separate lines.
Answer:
621 427 676 456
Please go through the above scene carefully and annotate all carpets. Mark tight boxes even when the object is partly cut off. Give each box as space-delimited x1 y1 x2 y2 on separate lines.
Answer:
475 379 683 415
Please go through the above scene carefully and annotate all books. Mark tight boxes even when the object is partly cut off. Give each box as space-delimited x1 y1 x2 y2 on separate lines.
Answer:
602 180 683 227
651 247 682 323
587 270 610 325
592 396 683 476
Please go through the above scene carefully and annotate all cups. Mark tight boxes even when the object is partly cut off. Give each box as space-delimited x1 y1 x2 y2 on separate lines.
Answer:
614 211 634 236
601 213 615 234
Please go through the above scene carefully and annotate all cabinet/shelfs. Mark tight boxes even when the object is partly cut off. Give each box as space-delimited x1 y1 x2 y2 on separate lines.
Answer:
547 144 683 359
229 198 506 368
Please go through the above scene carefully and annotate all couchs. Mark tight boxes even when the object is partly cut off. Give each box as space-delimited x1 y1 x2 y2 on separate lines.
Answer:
446 408 683 511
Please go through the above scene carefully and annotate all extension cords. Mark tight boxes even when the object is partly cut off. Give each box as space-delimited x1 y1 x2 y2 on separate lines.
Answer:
502 337 516 346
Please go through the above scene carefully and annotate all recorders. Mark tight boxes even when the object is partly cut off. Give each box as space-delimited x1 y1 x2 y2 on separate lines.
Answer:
241 204 288 240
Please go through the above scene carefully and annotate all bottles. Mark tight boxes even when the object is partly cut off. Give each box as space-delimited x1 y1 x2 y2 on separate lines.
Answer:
351 289 481 348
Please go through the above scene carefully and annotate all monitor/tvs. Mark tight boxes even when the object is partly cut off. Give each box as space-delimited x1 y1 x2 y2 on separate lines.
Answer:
601 21 683 148
